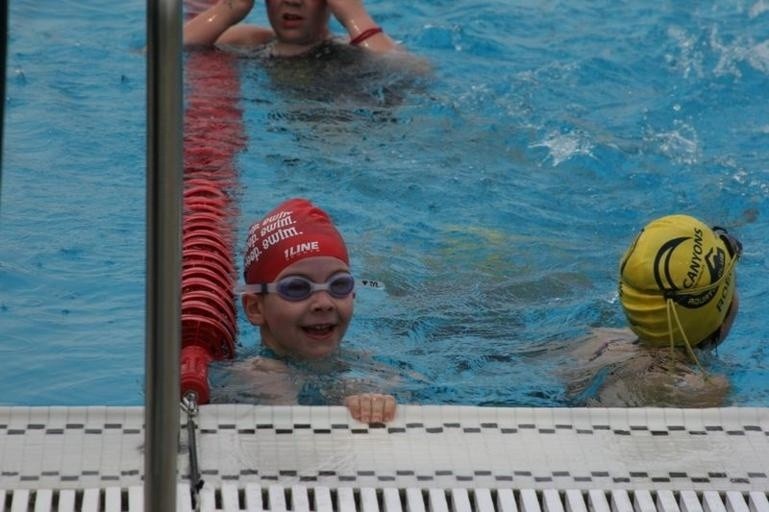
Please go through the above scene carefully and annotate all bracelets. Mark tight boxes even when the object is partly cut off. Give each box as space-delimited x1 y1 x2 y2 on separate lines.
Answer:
347 25 385 49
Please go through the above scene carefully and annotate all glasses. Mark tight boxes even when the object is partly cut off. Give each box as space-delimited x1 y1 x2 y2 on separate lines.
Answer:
232 275 386 301
712 227 741 260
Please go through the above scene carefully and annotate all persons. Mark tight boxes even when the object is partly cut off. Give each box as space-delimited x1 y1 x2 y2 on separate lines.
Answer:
219 196 430 424
565 212 744 407
182 0 393 54
